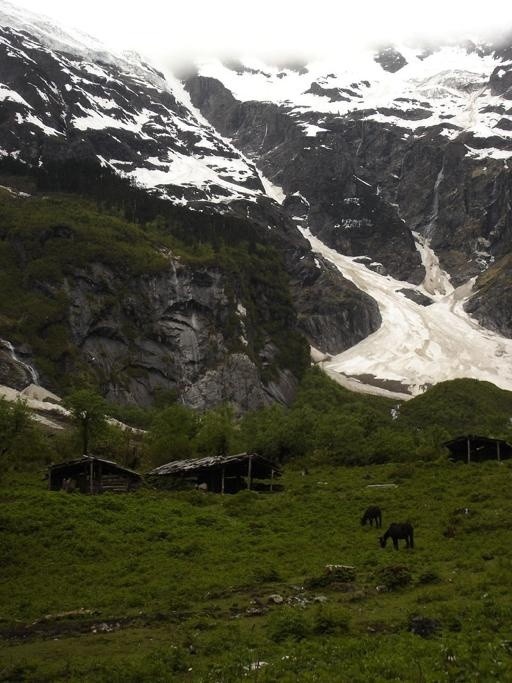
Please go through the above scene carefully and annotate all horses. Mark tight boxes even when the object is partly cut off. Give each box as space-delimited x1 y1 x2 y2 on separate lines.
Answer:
359 505 383 529
377 522 414 550
